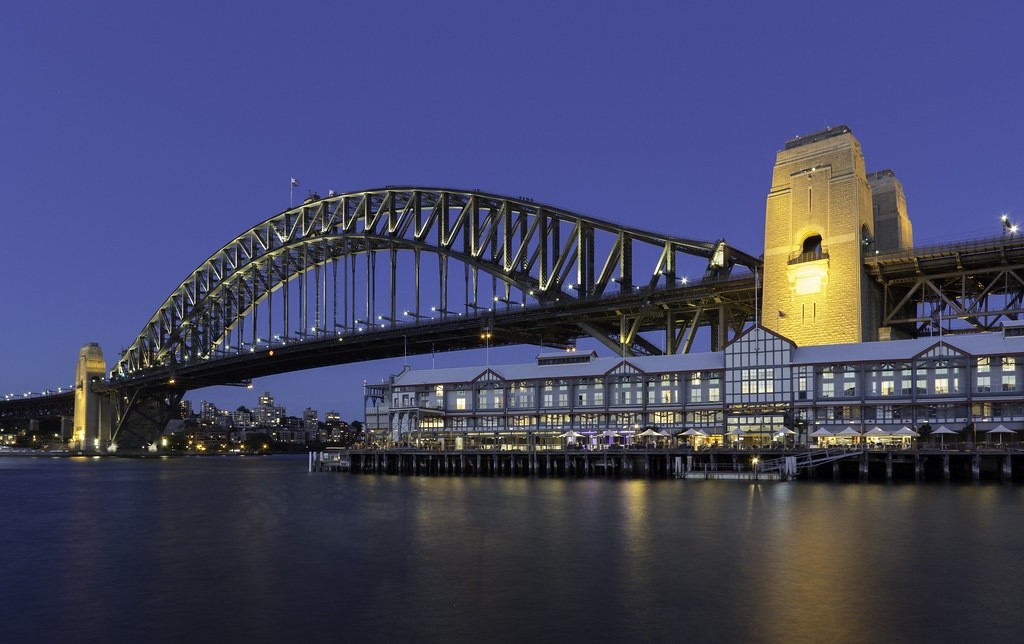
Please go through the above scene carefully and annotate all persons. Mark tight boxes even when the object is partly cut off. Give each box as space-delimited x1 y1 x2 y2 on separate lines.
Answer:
715 438 718 446
651 438 690 448
568 441 582 448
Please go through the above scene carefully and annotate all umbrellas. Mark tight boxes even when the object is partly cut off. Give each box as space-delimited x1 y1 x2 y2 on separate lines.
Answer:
837 427 861 445
698 429 709 444
929 425 959 450
863 427 890 449
597 429 622 448
987 425 1018 444
639 429 663 443
659 430 671 441
558 430 584 448
809 428 834 448
724 428 747 448
775 427 797 450
677 428 703 448
892 426 920 447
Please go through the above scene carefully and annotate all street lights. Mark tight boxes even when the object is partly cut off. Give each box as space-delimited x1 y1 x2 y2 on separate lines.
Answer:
290 176 299 208
